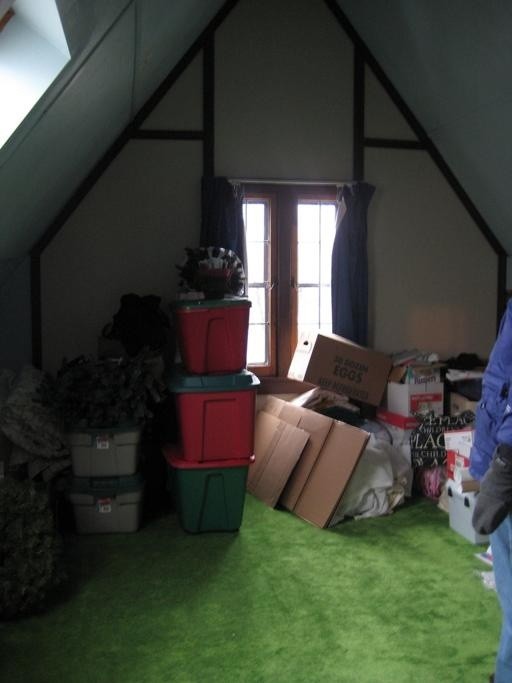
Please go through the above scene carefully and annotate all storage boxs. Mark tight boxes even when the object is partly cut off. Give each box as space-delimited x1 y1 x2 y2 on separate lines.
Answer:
444 424 483 473
387 381 444 418
401 352 446 383
288 330 393 405
174 296 253 374
69 419 144 477
68 477 147 534
449 383 493 430
166 372 262 460
156 458 256 532
447 480 492 545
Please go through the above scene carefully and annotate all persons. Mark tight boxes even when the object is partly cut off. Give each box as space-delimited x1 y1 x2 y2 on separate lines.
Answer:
467 288 512 681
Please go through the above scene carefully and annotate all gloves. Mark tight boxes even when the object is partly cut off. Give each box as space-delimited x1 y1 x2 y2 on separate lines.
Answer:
472 444 510 534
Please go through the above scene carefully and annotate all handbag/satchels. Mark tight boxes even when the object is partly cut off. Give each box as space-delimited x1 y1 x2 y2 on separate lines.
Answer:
411 410 474 469
